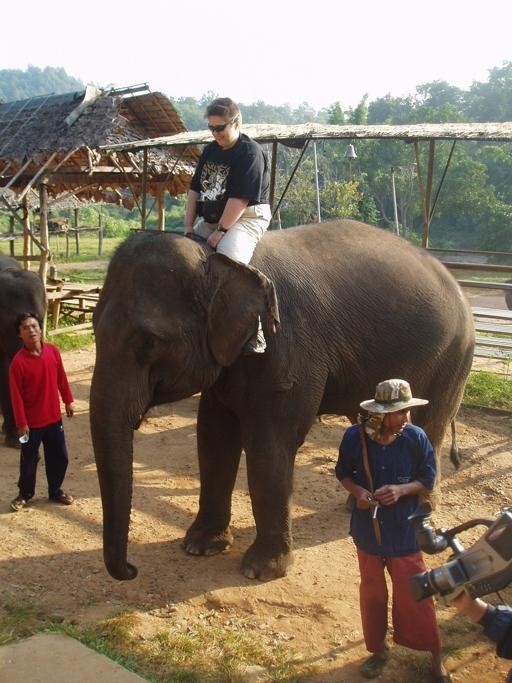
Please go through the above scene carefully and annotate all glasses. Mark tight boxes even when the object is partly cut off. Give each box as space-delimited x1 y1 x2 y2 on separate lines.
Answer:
207 113 240 132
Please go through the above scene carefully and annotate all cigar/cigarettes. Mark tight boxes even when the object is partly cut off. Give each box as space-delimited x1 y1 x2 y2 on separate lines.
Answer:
371 502 379 517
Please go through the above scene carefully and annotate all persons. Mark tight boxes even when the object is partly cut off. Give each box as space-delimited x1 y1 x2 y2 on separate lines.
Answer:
186 96 272 355
452 591 510 682
10 311 77 510
337 377 453 682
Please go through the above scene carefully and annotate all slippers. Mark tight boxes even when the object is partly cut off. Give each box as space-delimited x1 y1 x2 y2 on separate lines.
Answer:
49 492 74 504
9 495 33 511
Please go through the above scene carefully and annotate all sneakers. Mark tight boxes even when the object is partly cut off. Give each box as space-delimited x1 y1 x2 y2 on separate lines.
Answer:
359 642 393 679
429 664 453 683
243 331 268 354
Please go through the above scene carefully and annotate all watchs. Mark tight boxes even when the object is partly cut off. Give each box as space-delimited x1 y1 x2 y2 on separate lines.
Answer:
216 223 228 233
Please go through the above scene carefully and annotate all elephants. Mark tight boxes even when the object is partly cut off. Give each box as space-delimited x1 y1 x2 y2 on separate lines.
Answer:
0 250 47 449
89 217 477 584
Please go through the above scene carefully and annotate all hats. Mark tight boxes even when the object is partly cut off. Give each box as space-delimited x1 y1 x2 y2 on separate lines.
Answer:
359 377 430 415
